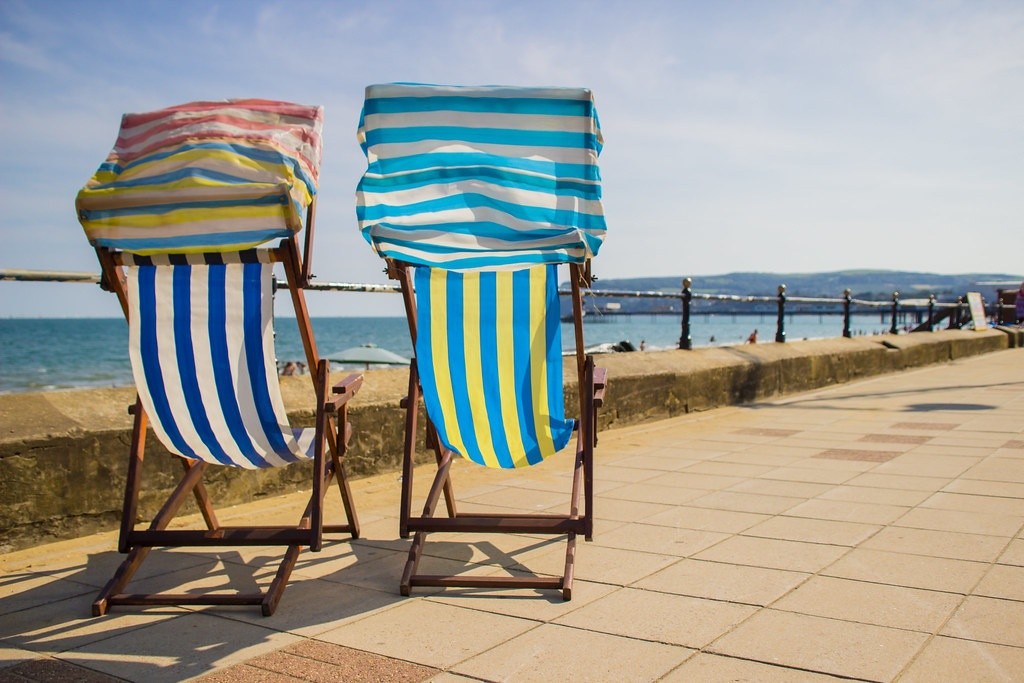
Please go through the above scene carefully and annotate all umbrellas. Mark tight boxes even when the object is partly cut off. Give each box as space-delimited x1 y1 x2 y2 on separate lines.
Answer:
320 343 412 370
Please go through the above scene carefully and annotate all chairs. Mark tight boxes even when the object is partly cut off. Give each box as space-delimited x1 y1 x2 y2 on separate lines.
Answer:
354 80 610 602
69 98 365 617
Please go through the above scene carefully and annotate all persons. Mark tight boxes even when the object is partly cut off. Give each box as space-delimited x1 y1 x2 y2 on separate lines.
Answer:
282 361 306 376
747 329 759 344
709 335 715 345
640 340 646 351
